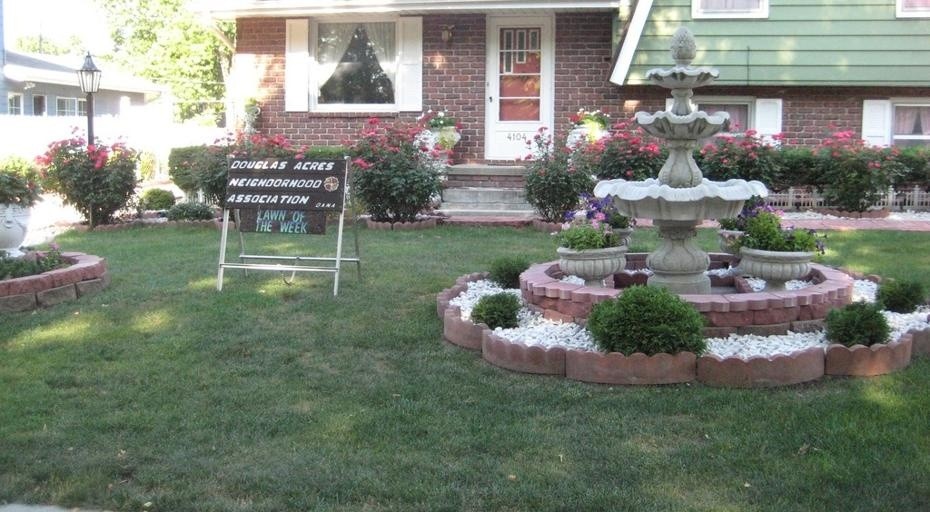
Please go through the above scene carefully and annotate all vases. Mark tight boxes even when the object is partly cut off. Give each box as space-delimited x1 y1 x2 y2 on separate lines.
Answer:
612 228 633 246
429 126 461 182
556 246 628 288
717 229 745 259
566 124 610 154
738 245 814 292
0 201 32 257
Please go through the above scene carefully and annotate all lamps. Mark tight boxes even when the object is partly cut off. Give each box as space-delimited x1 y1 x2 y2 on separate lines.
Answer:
438 22 455 42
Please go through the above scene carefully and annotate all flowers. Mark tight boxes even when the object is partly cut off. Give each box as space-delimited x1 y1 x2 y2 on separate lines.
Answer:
0 155 45 207
553 191 630 252
718 194 828 257
568 106 611 129
415 109 462 130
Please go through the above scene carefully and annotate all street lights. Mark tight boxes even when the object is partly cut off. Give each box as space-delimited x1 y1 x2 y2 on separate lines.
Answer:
74 51 106 229
